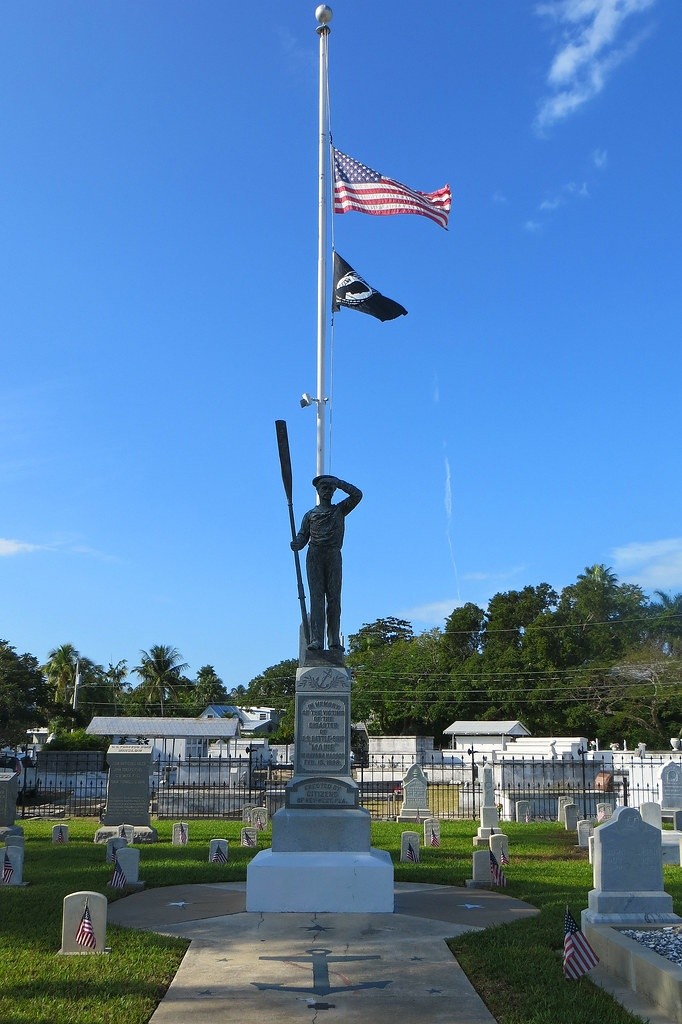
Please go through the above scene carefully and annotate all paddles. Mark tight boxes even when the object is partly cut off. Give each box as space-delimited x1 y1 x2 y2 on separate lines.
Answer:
275 419 311 647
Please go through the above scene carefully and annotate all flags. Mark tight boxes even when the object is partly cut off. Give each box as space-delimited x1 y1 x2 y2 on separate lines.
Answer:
56 826 64 844
212 845 228 864
74 904 96 950
406 843 419 863
111 860 128 889
330 142 451 232
331 250 408 322
500 845 509 864
562 909 600 980
488 847 506 887
111 845 117 863
244 832 255 847
1 851 14 883
180 823 186 844
120 827 127 838
414 810 420 823
256 817 263 831
430 829 439 846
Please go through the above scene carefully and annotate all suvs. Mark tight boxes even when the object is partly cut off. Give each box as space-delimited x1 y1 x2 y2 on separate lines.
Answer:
1 753 41 804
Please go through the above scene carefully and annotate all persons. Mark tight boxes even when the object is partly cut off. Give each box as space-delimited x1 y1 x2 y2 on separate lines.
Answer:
291 475 363 652
